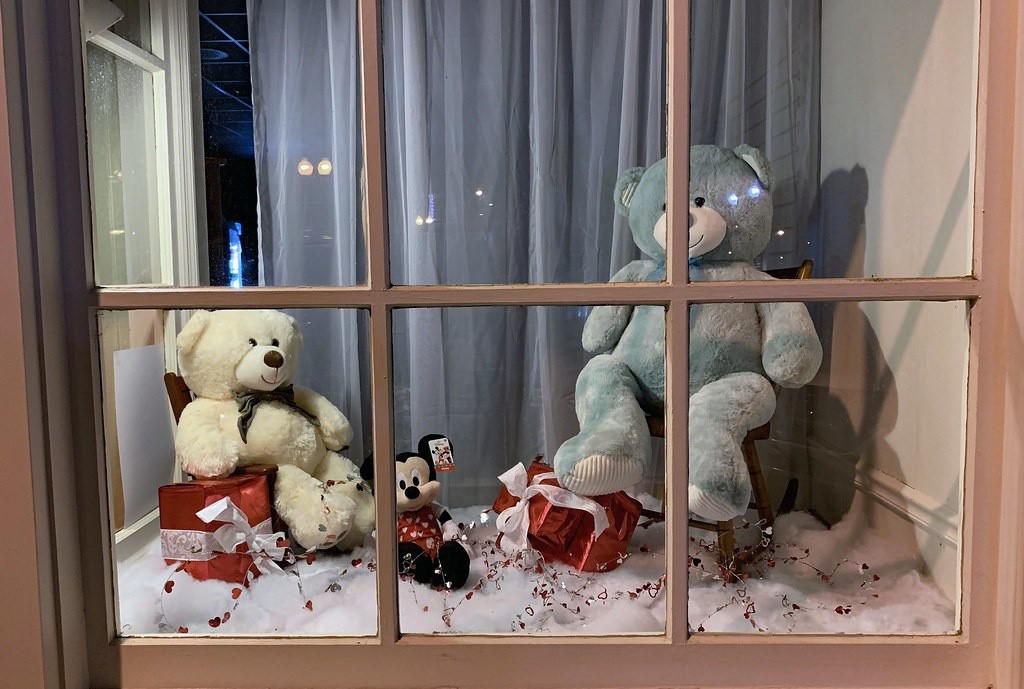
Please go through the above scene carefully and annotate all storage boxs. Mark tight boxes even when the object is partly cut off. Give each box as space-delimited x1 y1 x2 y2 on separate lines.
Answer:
157 474 287 589
495 462 643 573
492 461 611 557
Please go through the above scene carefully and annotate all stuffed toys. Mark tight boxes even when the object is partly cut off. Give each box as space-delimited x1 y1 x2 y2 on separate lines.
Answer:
554 144 824 520
358 434 473 590
176 309 377 554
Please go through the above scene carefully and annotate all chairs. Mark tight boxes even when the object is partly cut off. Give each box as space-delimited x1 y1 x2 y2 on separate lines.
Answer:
638 259 816 583
164 371 300 553
